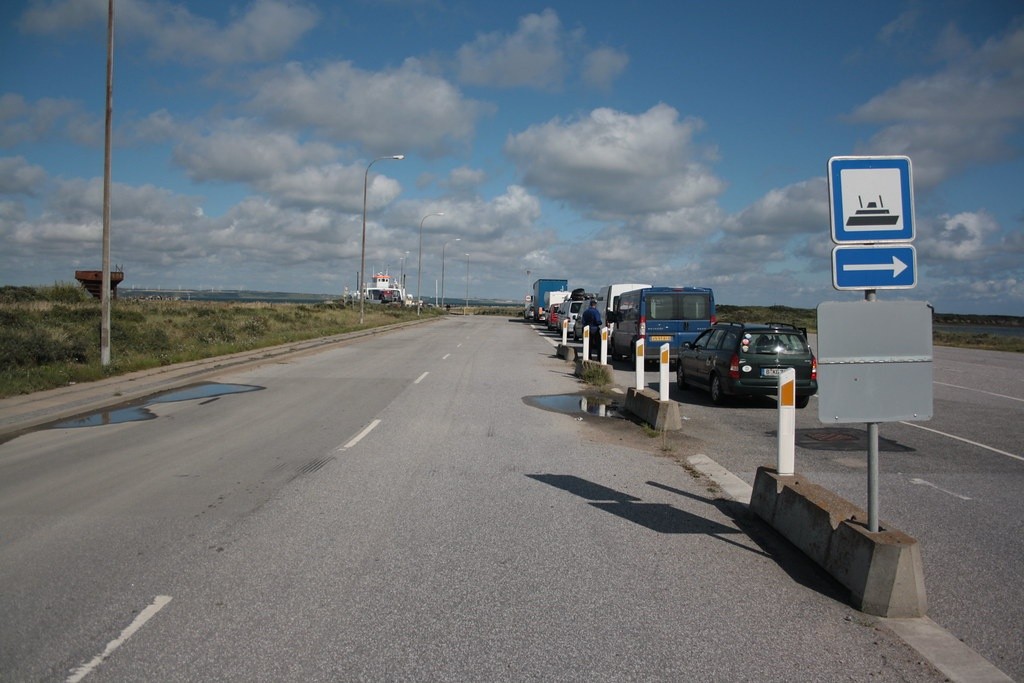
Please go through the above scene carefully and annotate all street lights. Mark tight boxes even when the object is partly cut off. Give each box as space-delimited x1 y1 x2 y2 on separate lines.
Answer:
417 212 444 318
441 238 462 310
359 155 404 325
464 253 470 307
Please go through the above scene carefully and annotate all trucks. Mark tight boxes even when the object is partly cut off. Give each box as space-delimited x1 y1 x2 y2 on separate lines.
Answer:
596 284 654 343
532 278 572 322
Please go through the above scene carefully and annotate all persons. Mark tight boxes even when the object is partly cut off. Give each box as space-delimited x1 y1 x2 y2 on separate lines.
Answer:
582 299 603 362
446 303 451 315
545 310 549 325
542 308 545 317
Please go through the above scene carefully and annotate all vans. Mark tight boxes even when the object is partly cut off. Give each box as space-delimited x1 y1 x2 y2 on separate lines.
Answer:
606 287 717 367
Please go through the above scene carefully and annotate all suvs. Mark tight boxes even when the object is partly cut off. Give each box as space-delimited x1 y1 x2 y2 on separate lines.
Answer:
523 304 534 319
675 319 818 409
545 300 595 340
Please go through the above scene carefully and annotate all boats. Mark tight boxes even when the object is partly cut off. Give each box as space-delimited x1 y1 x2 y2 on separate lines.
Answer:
334 268 424 307
